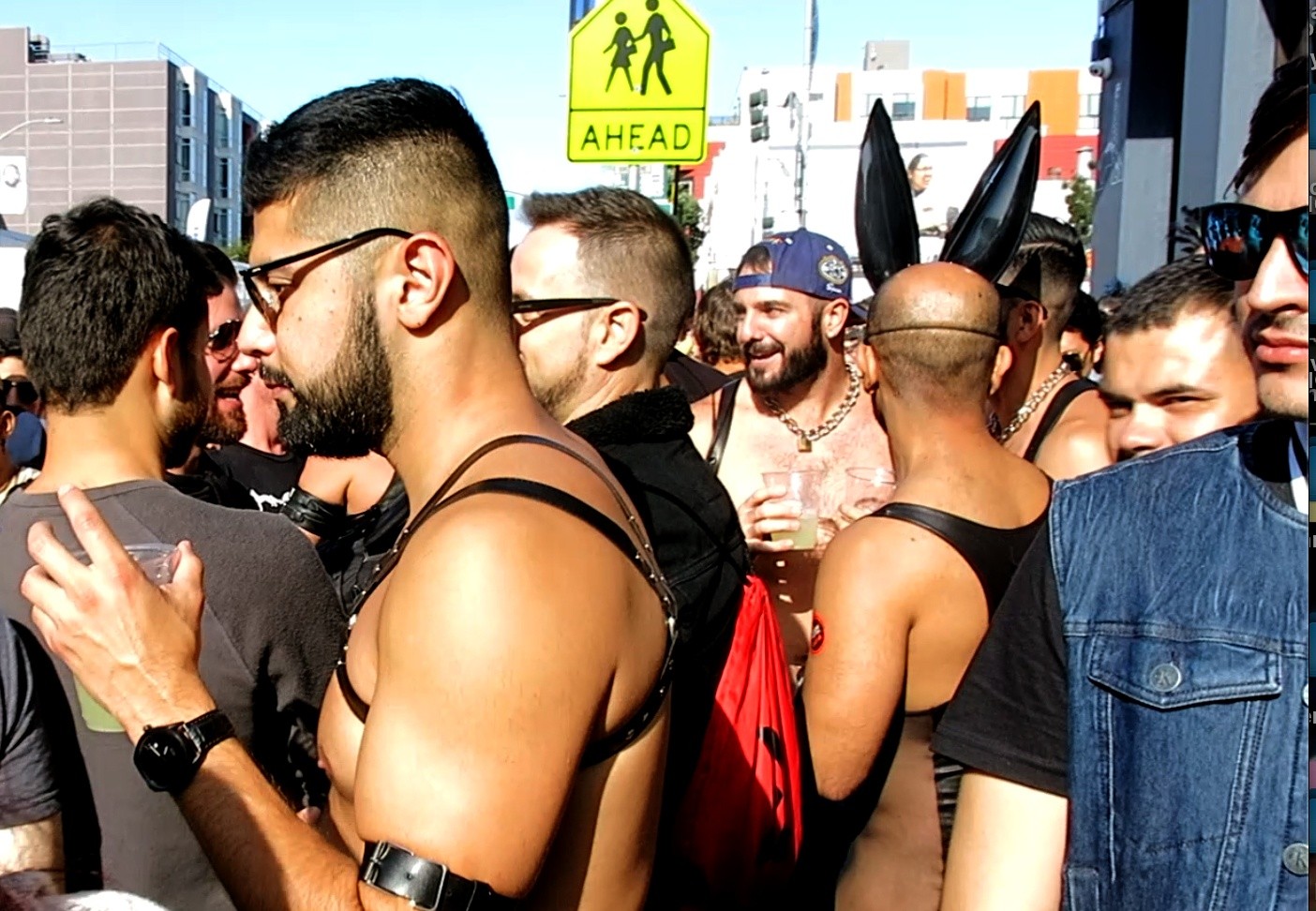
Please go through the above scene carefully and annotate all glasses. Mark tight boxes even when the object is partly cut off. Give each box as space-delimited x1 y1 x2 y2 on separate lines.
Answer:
0 380 39 405
1062 347 1095 372
1191 202 1310 280
238 227 415 315
207 320 242 350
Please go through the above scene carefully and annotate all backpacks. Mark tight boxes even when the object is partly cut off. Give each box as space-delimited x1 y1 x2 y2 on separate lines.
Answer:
628 479 805 893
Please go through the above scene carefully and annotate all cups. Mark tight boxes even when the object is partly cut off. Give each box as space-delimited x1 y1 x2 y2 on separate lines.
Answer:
62 544 181 732
761 468 825 549
835 468 896 532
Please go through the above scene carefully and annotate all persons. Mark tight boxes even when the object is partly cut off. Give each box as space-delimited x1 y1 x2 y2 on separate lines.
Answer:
758 360 861 453
930 50 1316 911
907 149 943 264
689 228 899 697
1 199 1315 911
793 98 1061 911
1096 251 1260 463
510 185 756 911
19 77 683 910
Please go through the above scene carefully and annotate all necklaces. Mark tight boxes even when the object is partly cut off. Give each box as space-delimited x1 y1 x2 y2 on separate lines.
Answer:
987 362 1070 444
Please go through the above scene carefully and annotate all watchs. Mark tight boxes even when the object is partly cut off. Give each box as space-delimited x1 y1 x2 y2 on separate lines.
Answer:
132 707 237 793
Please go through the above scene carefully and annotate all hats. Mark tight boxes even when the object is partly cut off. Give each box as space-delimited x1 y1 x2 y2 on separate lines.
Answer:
732 227 868 327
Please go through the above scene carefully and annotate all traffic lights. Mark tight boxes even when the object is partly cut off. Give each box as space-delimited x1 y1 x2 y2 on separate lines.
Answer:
748 90 770 143
